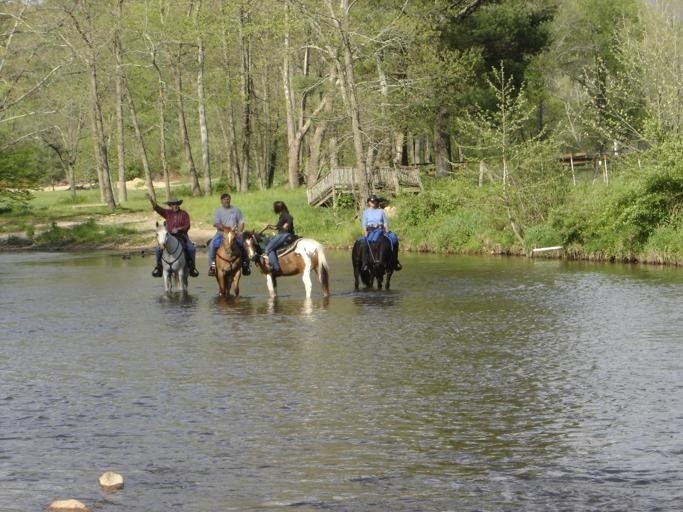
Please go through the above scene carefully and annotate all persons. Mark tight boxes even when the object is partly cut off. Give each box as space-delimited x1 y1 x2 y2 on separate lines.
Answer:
264 200 297 278
144 191 200 278
208 193 252 276
360 194 402 277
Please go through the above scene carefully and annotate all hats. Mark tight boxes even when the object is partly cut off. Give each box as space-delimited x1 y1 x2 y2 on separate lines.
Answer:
161 195 183 205
367 194 384 203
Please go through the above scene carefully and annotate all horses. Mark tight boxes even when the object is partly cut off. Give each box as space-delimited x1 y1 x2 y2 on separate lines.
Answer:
369 232 402 290
206 223 242 304
236 228 330 299
155 220 196 298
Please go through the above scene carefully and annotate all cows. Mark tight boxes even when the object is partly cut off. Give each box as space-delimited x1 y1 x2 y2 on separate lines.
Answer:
351 238 375 289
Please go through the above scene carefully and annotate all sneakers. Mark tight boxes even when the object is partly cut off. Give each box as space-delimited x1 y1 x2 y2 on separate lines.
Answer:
272 271 281 276
188 263 198 276
152 262 161 277
208 268 214 274
